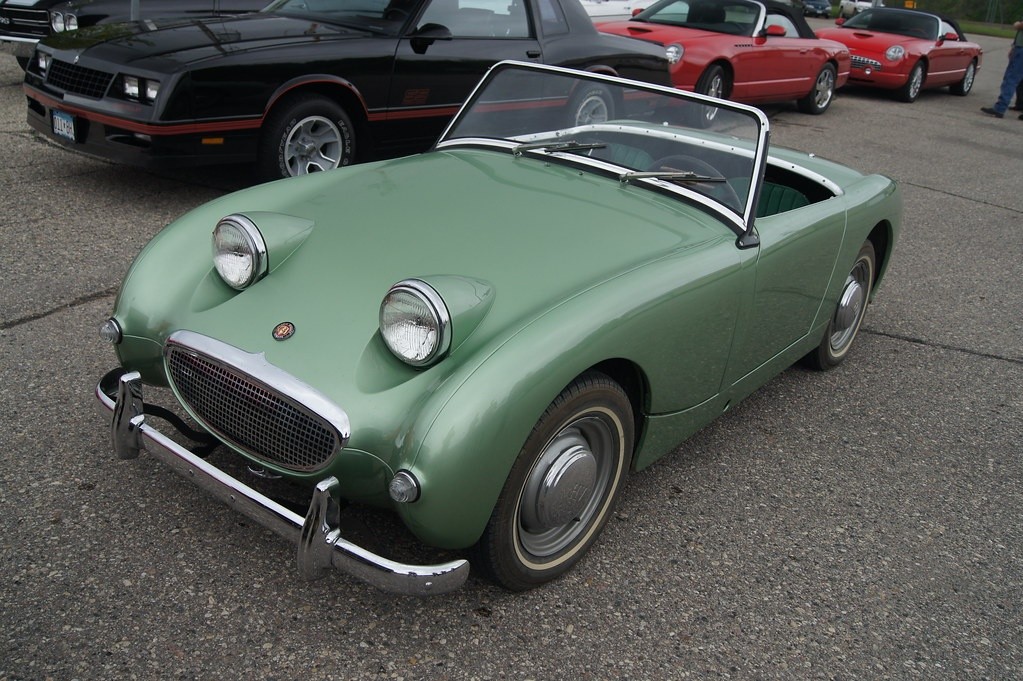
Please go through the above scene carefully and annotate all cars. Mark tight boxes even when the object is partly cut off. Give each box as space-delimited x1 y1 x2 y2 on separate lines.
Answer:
0 0 675 182
792 0 833 20
839 0 886 21
89 58 907 599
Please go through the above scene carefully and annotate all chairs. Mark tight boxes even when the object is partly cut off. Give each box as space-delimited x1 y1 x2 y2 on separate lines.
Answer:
699 5 726 24
581 140 655 171
709 178 811 218
452 7 494 36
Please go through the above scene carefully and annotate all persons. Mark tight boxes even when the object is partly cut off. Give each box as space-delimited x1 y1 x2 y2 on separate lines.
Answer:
1008 78 1023 121
980 22 1023 118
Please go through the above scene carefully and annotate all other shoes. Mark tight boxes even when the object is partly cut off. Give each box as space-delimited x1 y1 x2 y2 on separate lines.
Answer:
1008 106 1019 111
1018 114 1023 121
981 107 1003 118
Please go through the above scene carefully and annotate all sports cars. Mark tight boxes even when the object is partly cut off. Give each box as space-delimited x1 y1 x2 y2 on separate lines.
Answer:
590 0 851 116
813 6 983 103
